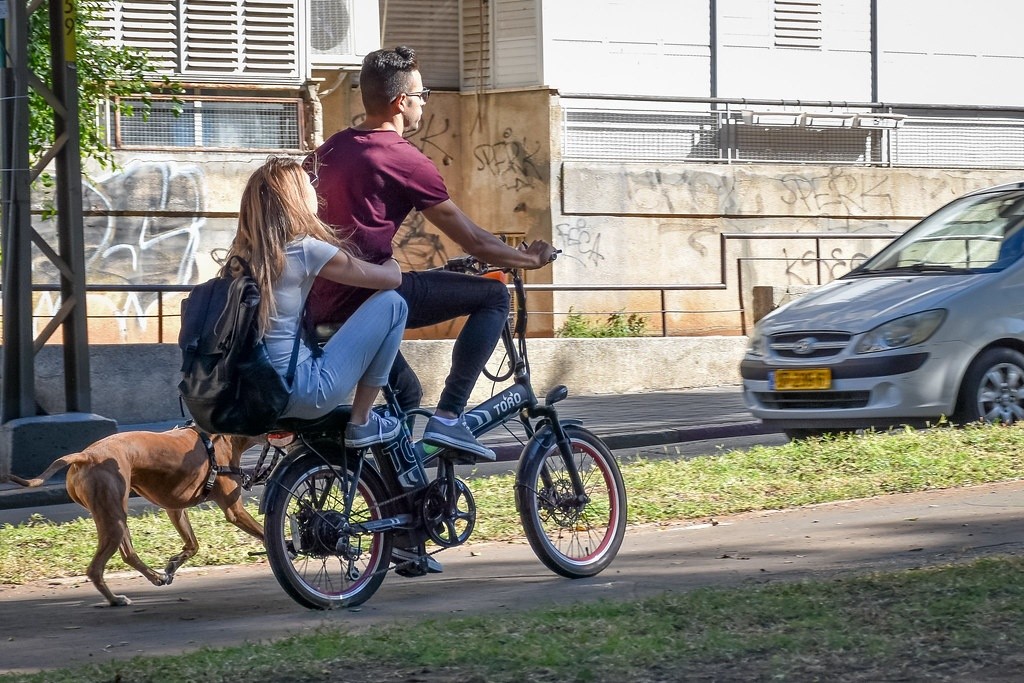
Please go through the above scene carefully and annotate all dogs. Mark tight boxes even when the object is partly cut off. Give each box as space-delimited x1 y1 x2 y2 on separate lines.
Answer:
5 417 272 608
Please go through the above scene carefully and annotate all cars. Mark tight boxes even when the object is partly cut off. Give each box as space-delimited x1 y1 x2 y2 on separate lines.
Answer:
740 180 1024 441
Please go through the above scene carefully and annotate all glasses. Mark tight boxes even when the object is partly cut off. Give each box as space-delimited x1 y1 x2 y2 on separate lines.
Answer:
390 86 431 103
305 171 320 190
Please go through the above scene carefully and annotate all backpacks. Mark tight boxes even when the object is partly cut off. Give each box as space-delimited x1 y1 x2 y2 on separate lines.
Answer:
178 254 309 440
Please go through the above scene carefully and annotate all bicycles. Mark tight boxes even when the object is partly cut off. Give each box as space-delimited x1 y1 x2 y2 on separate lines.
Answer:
256 242 631 612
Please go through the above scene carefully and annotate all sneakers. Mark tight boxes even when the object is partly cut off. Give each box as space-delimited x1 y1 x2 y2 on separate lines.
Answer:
422 411 497 463
344 408 401 449
391 544 443 574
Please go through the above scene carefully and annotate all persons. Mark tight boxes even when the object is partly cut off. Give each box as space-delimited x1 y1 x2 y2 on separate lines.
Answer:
209 41 559 574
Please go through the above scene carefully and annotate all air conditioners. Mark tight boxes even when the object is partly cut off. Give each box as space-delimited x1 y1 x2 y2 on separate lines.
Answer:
310 2 384 73
